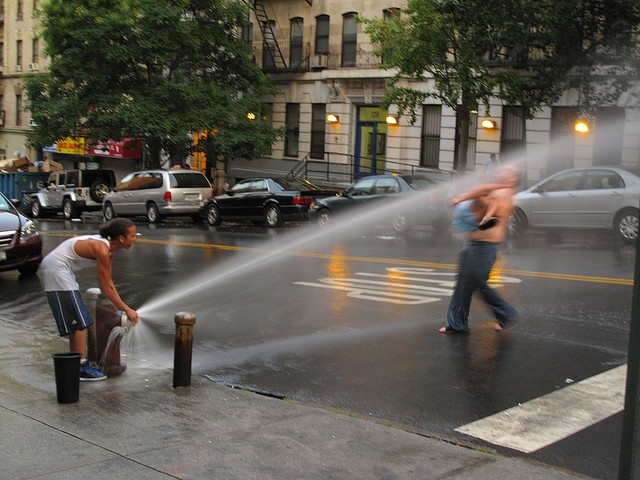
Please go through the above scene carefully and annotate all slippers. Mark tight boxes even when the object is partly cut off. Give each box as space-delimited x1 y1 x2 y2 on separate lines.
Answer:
438 324 454 333
494 319 520 332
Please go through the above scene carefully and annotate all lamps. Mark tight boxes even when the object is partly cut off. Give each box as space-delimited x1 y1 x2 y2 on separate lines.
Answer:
574 115 590 137
245 108 259 125
327 113 341 129
385 111 399 128
480 117 496 132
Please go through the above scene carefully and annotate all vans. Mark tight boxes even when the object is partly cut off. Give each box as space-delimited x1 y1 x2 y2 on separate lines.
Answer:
101 168 214 224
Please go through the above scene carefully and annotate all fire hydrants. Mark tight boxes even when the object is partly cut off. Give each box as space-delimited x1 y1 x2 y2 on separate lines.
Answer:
95 296 127 377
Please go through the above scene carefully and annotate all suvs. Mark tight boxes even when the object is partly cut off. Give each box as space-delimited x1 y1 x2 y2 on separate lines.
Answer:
29 168 118 221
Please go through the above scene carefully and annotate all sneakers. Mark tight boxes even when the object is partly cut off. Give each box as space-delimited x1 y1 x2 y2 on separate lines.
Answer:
79 360 107 380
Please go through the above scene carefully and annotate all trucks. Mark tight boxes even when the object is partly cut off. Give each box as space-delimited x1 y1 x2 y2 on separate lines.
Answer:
1 171 47 209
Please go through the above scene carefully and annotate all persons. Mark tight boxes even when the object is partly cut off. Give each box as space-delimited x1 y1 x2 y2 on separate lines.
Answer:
448 183 504 235
37 218 140 382
438 165 525 338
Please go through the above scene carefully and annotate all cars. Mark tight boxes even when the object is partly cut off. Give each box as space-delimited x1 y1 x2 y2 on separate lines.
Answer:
306 171 455 237
0 191 45 278
196 176 341 229
504 165 640 246
